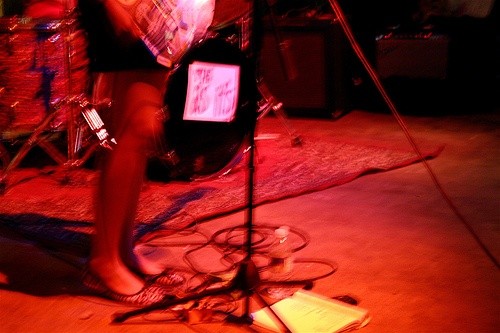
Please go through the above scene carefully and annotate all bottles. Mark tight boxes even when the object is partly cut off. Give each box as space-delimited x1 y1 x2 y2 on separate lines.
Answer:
266 226 295 300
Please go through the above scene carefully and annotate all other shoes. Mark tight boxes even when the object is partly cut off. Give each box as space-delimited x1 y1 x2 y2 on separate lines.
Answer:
78 262 185 307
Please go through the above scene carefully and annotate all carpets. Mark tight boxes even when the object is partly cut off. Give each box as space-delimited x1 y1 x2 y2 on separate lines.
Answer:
1 127 439 260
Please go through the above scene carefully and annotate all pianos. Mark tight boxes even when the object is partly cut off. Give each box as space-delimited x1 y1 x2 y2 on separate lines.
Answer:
372 30 449 89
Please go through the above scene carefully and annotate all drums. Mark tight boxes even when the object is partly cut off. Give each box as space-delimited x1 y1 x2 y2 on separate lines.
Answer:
130 1 217 67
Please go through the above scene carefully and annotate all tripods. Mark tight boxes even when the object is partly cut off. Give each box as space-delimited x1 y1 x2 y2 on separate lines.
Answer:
109 0 315 333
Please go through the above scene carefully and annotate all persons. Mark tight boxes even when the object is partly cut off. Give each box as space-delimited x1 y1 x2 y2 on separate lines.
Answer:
75 0 188 309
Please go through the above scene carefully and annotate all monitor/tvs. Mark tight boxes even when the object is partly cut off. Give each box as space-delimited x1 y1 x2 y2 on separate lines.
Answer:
181 60 240 123
